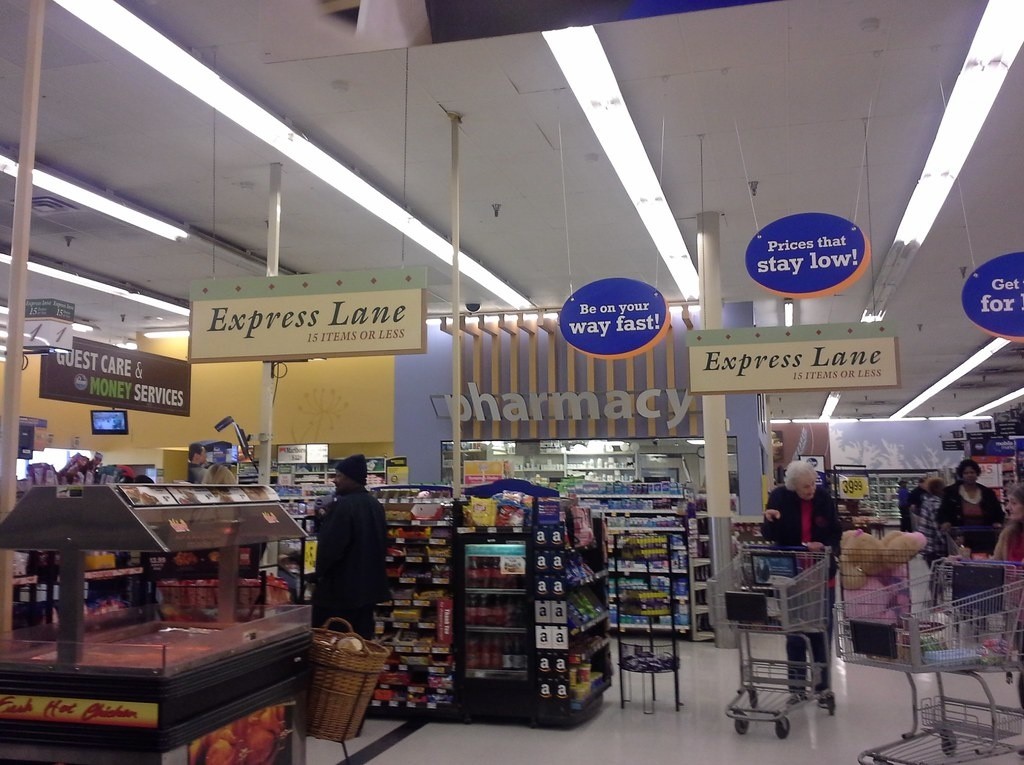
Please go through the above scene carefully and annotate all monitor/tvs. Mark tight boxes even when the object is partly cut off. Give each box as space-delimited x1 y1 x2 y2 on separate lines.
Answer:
90 409 128 435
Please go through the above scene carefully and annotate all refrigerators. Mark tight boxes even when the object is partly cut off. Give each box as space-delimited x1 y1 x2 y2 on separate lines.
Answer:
456 524 538 727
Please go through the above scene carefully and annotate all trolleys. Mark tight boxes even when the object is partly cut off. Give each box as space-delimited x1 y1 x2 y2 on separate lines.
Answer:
724 546 836 741
833 525 1024 765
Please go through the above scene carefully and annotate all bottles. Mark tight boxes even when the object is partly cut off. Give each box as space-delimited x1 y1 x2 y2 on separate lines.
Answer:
468 557 528 670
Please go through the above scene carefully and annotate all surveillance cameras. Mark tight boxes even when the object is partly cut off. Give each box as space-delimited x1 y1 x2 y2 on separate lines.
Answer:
465 300 481 312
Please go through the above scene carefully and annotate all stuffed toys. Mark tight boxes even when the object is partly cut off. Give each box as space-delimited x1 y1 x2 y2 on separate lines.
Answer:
841 528 927 624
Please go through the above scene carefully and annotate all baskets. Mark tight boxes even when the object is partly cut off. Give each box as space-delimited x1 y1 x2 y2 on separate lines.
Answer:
303 618 391 743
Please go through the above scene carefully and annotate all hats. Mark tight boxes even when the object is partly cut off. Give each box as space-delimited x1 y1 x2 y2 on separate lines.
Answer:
335 453 368 486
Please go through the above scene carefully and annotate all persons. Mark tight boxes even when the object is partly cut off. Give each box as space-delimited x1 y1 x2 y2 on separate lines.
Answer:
201 462 237 484
309 453 388 640
897 459 1005 638
189 444 207 483
760 461 842 707
945 486 1024 758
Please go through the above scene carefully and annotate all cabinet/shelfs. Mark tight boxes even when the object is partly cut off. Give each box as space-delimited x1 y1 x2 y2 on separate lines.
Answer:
10 480 702 742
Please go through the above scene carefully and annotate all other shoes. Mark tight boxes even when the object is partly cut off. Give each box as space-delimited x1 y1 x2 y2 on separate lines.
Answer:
785 694 808 707
817 694 829 709
